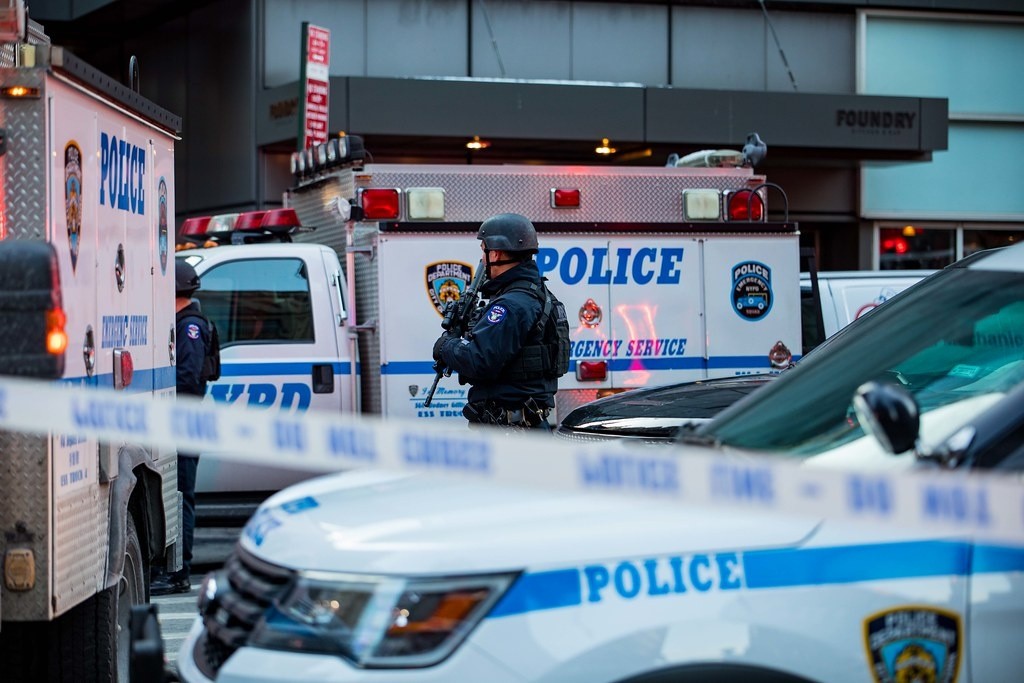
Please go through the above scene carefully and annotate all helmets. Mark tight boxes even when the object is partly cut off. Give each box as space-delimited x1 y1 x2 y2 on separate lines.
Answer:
175 259 200 293
478 213 538 255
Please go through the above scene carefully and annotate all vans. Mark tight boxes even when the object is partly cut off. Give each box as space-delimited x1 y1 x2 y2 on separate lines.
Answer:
802 269 1022 378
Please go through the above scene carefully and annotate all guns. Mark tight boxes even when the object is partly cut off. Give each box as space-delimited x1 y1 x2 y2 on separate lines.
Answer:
424 258 486 408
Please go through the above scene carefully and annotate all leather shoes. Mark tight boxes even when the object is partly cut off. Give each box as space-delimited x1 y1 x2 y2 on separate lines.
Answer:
152 574 190 594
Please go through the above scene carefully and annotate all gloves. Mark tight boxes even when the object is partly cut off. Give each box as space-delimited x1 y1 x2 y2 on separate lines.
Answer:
433 336 463 363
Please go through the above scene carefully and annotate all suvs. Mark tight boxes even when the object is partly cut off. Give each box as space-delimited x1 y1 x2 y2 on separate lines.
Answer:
176 238 1024 683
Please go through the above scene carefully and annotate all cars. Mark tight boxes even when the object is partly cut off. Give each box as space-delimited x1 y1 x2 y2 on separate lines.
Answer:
548 373 779 446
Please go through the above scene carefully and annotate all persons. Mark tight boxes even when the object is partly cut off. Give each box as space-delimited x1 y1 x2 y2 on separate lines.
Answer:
148 261 212 596
433 214 571 438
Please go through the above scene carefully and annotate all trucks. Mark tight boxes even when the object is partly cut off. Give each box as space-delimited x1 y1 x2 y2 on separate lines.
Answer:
177 130 801 528
0 1 187 683
